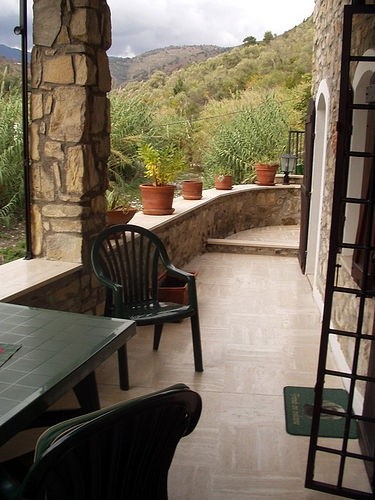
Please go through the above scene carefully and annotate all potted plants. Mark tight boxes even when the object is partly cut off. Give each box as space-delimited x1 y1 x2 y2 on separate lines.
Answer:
140 143 189 216
104 189 137 238
213 166 233 190
253 155 278 186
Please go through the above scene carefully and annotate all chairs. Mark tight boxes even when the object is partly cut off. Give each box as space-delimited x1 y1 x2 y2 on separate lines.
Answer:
90 225 203 391
0 383 202 500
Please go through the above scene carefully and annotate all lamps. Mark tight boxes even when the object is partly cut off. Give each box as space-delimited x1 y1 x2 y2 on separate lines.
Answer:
280 151 298 185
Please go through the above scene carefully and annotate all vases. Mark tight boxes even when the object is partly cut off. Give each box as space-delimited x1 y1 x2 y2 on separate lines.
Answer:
181 180 203 201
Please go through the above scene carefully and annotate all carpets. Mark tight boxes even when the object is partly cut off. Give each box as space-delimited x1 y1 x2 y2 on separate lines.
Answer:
283 386 360 440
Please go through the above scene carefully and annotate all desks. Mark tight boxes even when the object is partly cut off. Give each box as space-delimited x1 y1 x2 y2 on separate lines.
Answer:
0 301 137 446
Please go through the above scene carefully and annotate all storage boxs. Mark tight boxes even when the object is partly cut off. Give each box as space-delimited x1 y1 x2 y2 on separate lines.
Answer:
150 267 201 321
